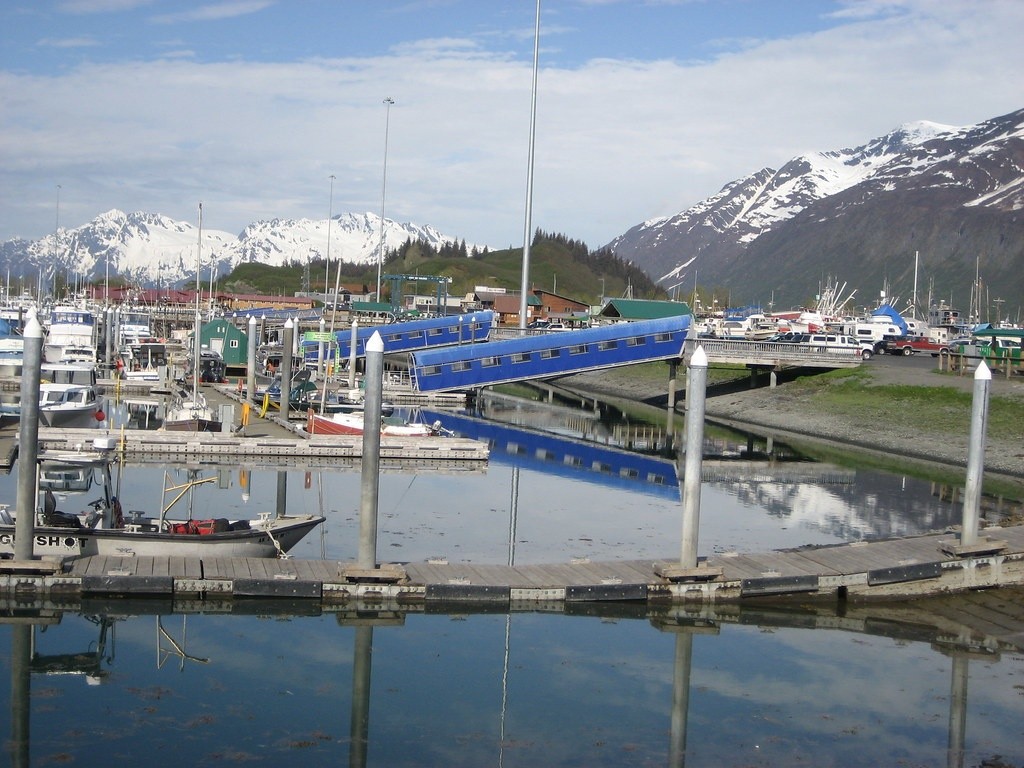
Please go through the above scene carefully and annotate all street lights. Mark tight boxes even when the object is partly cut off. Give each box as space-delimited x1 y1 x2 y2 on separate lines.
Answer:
375 95 394 318
324 174 337 309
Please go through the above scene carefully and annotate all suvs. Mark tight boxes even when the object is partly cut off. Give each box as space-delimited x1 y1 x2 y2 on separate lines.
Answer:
759 331 888 360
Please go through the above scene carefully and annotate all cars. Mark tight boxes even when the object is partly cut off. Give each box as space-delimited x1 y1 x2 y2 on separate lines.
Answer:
947 335 1022 353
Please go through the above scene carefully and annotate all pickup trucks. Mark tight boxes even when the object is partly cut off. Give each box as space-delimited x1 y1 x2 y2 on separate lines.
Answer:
886 336 951 356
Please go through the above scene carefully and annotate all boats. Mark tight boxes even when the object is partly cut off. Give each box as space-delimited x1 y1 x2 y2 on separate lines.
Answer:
689 269 780 340
0 470 326 560
0 254 167 388
18 363 106 428
237 371 355 414
307 407 457 438
0 376 23 422
